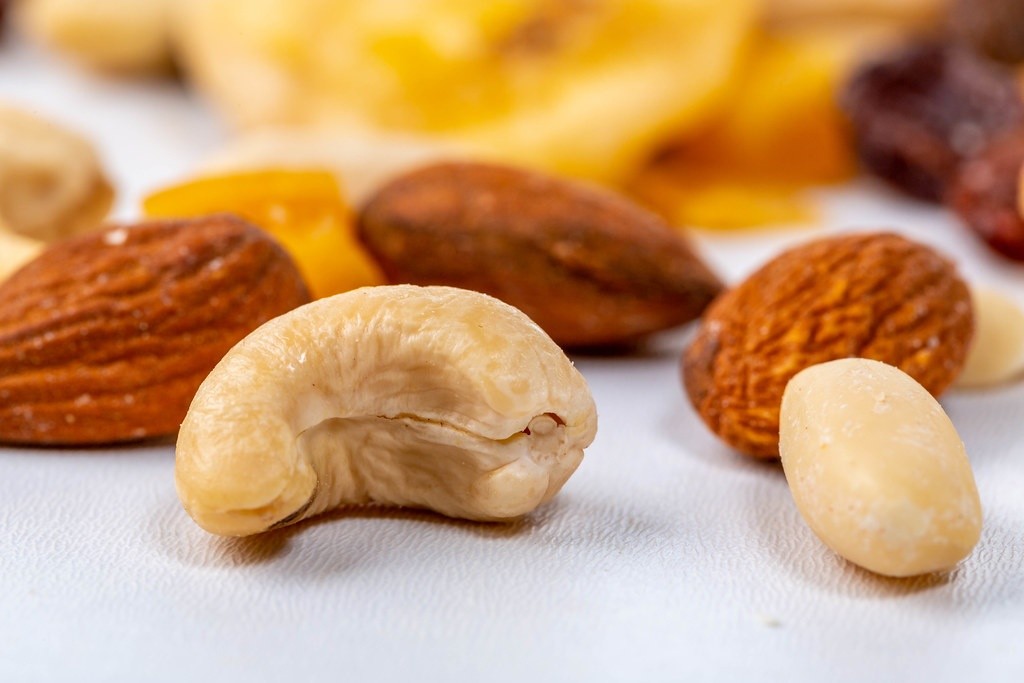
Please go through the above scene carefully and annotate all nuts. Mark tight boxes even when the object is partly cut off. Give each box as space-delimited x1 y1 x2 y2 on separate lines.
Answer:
0 0 1024 580
